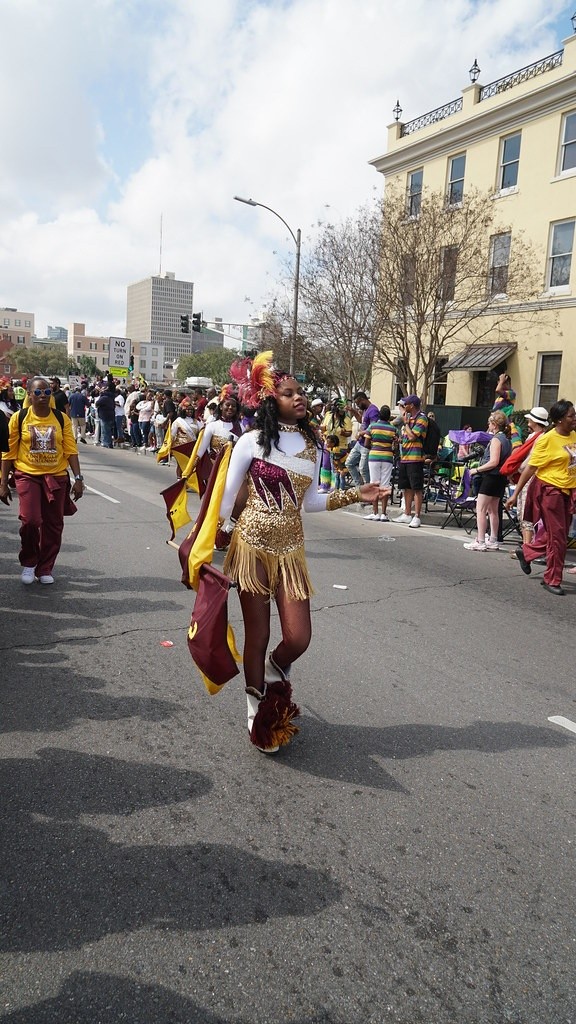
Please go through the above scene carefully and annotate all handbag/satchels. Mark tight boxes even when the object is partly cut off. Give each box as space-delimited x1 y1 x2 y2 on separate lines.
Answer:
154 414 167 429
472 474 481 495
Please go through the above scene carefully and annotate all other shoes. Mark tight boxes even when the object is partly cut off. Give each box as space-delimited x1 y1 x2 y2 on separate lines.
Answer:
318 489 327 494
380 514 390 521
81 438 87 444
409 517 420 527
543 583 564 595
392 513 412 523
515 547 531 575
363 513 379 520
567 567 576 574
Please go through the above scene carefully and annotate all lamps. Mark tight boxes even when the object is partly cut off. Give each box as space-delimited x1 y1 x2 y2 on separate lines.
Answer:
469 59 480 82
570 12 576 34
393 100 402 121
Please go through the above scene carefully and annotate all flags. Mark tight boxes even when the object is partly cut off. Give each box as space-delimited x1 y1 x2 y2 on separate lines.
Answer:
187 563 242 699
178 442 234 591
157 425 171 463
171 440 213 499
162 479 191 541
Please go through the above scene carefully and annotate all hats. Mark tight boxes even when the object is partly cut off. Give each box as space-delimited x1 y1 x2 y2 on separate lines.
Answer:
524 407 550 426
401 395 421 406
397 398 407 405
310 399 326 408
49 377 61 384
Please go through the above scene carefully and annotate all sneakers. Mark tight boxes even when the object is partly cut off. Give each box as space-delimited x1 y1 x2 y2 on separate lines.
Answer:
485 537 499 550
21 565 37 584
41 575 54 583
463 537 487 551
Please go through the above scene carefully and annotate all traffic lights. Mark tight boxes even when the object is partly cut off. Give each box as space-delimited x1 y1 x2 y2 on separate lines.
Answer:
180 314 190 334
192 311 203 333
128 355 134 371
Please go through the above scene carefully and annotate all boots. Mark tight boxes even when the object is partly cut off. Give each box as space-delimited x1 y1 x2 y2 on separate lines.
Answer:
263 649 300 744
245 683 281 754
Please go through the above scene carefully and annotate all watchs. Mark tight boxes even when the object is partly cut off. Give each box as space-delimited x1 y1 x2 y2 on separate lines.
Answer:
75 474 83 481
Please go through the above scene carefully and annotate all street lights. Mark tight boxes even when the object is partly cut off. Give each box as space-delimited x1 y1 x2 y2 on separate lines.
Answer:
232 194 302 376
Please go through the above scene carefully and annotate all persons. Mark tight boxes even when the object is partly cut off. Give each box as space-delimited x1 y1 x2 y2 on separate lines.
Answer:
0 376 83 583
505 398 576 594
213 373 357 754
0 370 571 551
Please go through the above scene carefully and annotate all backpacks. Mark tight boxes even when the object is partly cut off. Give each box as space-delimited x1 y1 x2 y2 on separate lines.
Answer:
413 415 440 456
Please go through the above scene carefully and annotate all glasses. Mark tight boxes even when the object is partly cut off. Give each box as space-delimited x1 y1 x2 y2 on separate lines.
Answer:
30 388 51 396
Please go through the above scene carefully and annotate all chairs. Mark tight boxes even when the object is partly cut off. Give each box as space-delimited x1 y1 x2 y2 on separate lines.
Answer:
434 461 523 542
